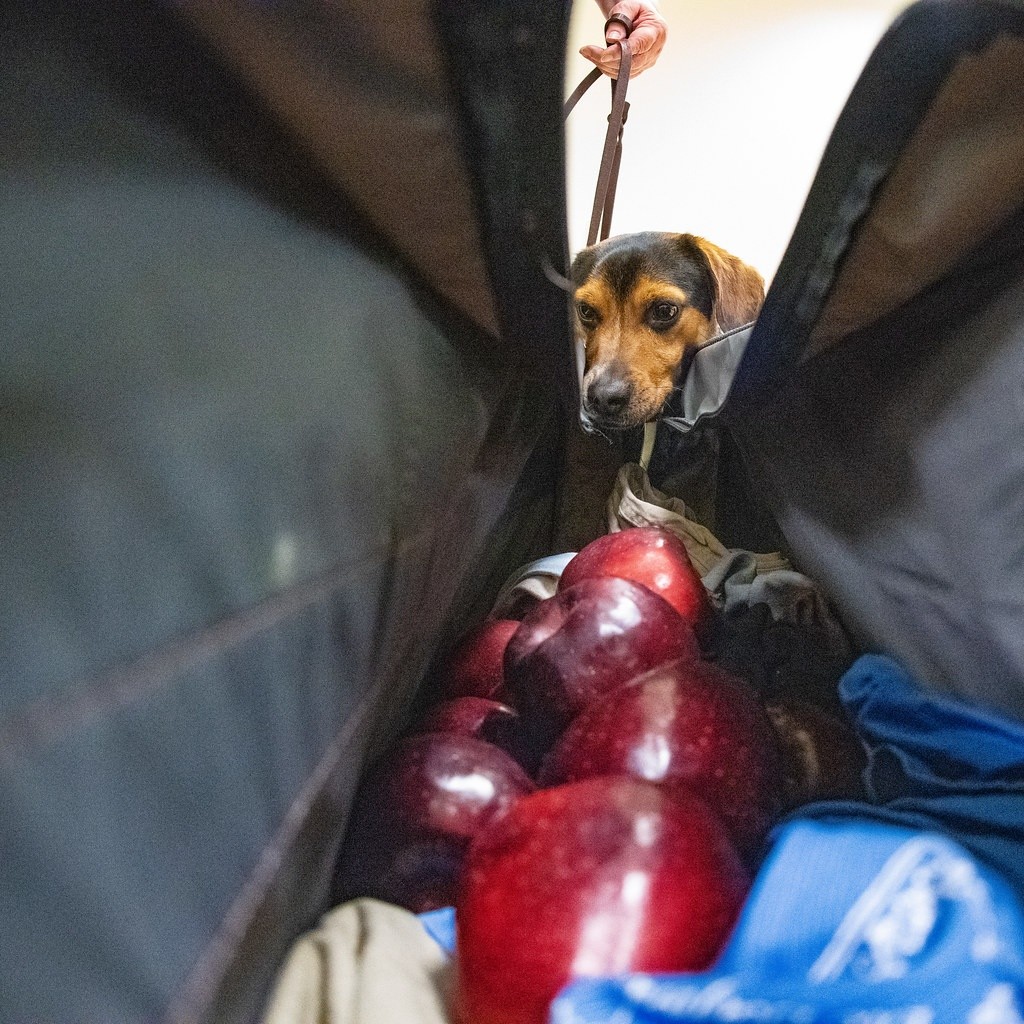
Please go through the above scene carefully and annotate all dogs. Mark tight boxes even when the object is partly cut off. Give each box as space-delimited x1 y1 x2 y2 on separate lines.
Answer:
569 232 766 447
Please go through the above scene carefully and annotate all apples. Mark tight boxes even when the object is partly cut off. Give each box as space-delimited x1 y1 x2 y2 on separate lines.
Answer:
330 516 865 1022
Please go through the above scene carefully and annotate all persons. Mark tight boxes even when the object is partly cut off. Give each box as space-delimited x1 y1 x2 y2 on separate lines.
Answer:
578 1 671 81
